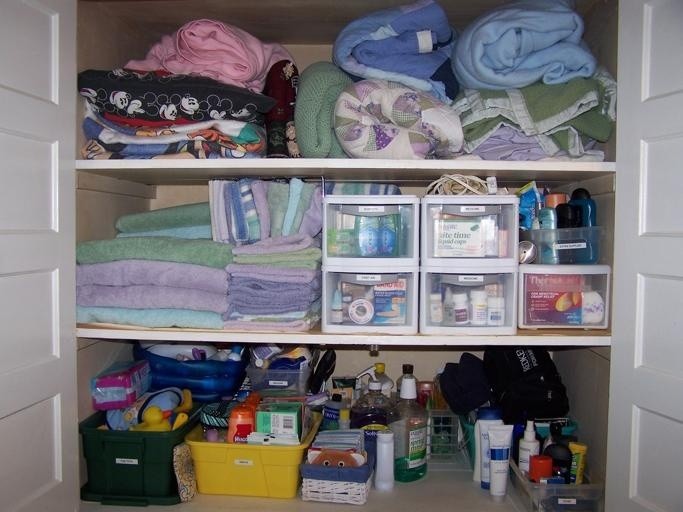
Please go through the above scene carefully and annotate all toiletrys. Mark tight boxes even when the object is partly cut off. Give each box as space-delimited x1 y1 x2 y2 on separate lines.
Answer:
473 407 502 482
330 387 352 400
488 425 514 497
332 376 356 388
478 417 504 488
539 206 558 262
478 418 588 503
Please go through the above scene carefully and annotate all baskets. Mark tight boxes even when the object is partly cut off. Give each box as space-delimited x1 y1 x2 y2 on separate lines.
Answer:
426 414 459 464
299 439 378 506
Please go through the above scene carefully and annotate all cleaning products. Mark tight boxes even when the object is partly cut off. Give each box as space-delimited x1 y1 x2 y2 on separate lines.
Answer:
349 363 399 462
363 363 395 395
519 420 540 476
321 394 347 430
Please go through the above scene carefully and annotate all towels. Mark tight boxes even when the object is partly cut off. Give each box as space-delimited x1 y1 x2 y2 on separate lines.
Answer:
326 178 399 195
76 177 322 331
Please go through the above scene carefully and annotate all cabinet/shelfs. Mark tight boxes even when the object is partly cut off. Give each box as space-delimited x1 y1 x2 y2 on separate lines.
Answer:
0 1 682 511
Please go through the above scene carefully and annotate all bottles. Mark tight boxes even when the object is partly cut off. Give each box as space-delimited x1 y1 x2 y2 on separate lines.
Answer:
374 430 396 492
452 293 469 325
517 419 540 474
430 293 442 323
487 297 505 326
320 393 351 429
396 363 419 393
538 186 600 265
470 290 488 325
367 362 394 398
205 389 260 444
348 380 396 428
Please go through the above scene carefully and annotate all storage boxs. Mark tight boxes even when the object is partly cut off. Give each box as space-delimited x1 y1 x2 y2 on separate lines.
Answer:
504 447 604 511
419 269 519 338
80 344 371 506
517 266 609 335
421 191 522 268
520 225 608 265
321 191 420 267
321 267 419 336
